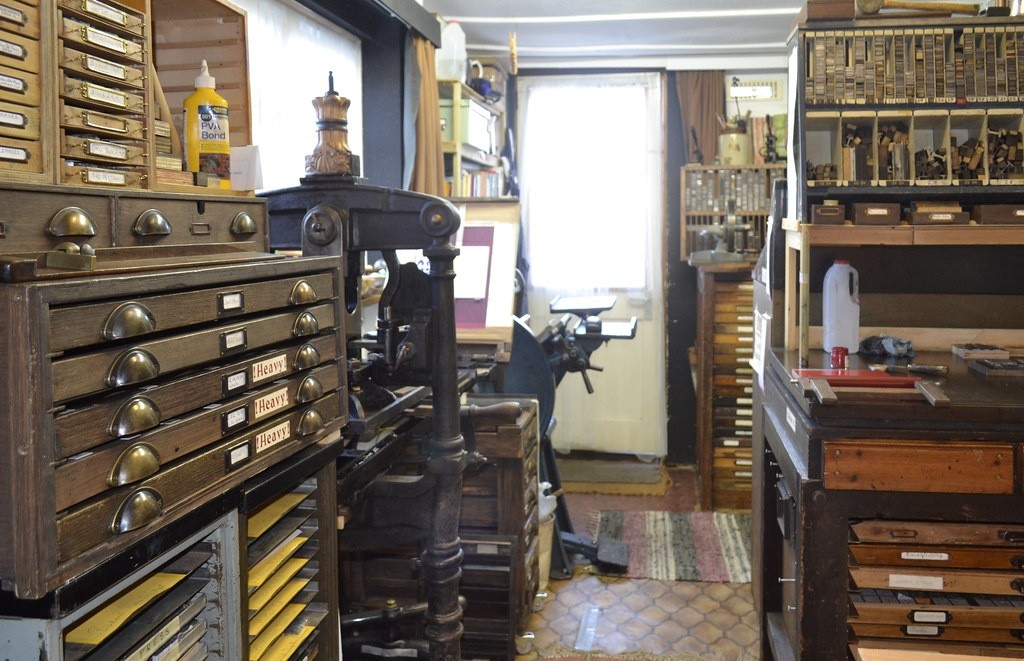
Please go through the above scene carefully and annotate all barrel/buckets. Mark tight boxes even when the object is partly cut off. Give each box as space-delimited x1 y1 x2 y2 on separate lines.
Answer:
539 512 555 590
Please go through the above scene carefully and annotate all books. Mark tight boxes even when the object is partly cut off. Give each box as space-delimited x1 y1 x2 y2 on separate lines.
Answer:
445 165 503 198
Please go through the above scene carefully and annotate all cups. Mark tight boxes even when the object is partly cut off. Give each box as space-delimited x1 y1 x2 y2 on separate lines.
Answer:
717 133 754 165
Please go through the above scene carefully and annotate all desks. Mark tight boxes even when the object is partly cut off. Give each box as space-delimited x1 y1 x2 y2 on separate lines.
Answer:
683 259 756 511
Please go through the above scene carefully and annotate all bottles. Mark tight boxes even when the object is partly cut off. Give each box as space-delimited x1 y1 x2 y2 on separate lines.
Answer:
830 347 849 369
434 20 466 82
823 259 860 354
182 59 231 190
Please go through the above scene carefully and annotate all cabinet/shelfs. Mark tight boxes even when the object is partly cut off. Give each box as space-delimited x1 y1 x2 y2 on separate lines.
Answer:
759 344 1024 661
0 0 349 661
437 77 507 198
340 393 540 661
785 15 1024 226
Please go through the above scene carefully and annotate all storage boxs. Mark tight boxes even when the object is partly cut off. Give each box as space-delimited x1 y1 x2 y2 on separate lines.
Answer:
472 66 506 97
439 98 492 154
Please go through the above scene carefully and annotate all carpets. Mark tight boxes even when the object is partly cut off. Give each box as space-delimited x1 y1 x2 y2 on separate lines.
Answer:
578 509 753 584
